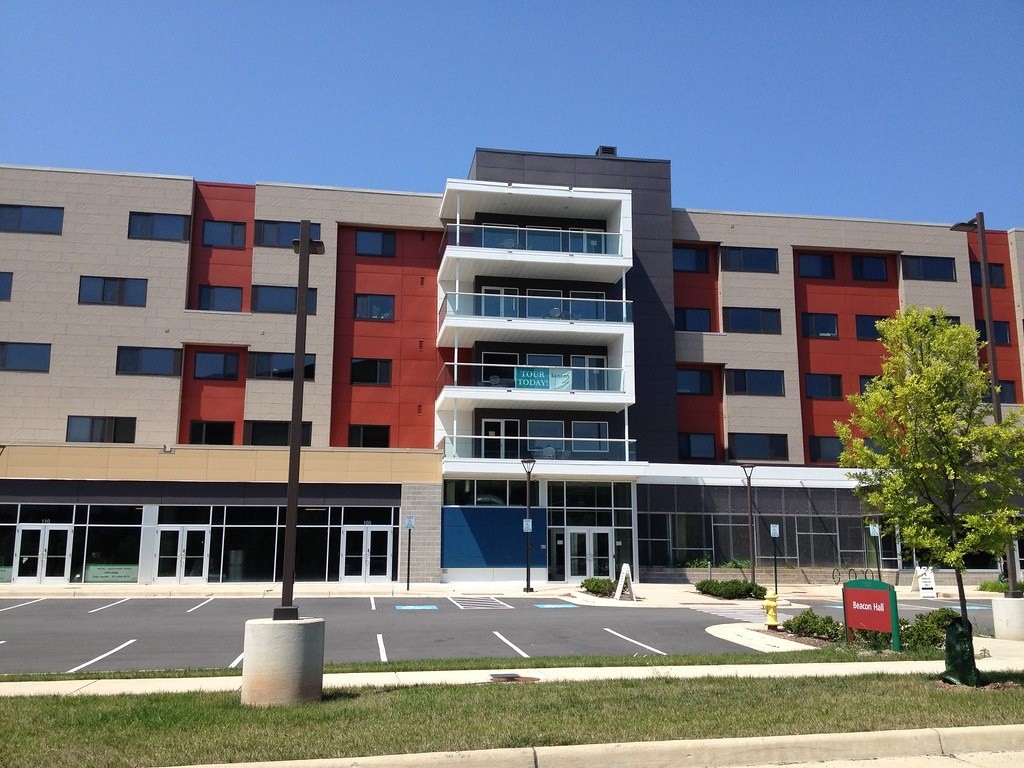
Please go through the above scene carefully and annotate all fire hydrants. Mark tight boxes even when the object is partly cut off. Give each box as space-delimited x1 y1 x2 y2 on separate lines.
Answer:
763 591 781 631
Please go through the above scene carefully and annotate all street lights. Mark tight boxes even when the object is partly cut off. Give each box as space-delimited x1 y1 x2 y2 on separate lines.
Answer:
521 451 537 592
242 219 326 709
742 463 757 584
951 211 1024 642
